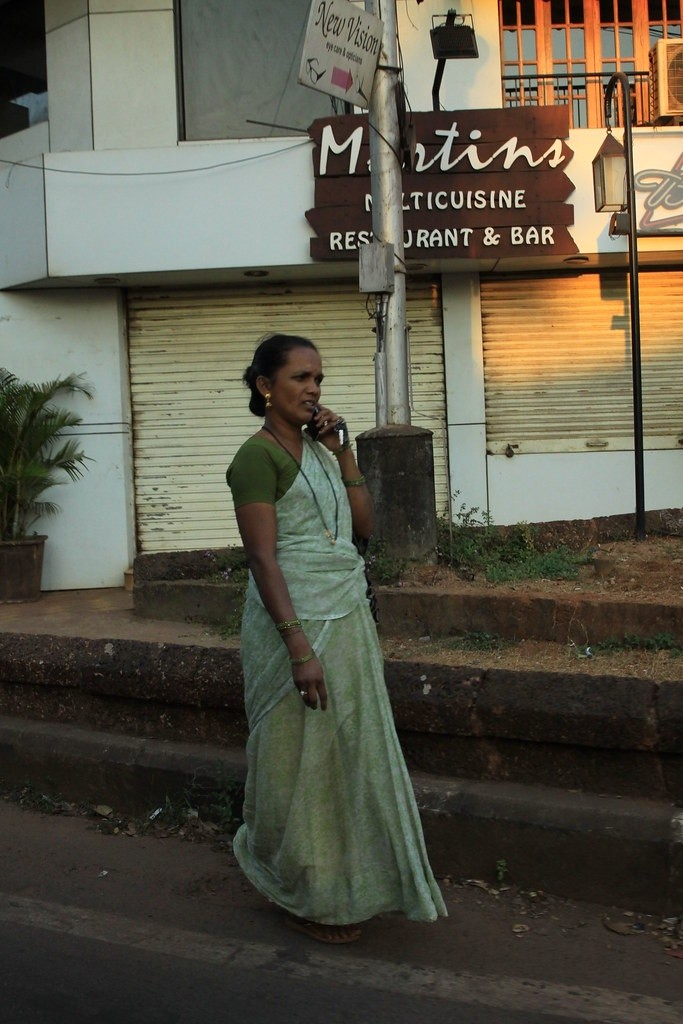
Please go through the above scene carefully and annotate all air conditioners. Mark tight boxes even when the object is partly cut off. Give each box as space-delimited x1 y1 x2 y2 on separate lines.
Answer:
648 37 683 127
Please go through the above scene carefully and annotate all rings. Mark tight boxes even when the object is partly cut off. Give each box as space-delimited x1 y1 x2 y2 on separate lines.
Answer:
337 416 344 424
300 691 307 696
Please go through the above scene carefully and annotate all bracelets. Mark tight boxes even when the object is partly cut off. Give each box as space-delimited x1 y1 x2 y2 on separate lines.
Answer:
289 648 316 666
276 620 301 630
339 472 366 487
332 443 354 455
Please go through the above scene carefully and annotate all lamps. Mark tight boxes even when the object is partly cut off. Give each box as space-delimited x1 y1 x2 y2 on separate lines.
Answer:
430 9 480 111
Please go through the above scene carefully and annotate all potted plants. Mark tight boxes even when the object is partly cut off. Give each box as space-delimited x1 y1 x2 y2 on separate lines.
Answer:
0 365 98 605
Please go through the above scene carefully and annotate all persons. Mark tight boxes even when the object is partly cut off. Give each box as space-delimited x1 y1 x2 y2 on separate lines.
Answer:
225 334 391 946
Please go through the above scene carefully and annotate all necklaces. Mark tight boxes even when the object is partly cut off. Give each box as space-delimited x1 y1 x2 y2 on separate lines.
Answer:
260 423 339 545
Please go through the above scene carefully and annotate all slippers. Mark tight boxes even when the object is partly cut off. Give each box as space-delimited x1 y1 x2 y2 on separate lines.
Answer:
285 911 362 944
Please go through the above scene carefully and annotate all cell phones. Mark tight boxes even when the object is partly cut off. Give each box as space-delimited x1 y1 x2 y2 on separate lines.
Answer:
308 407 323 442
